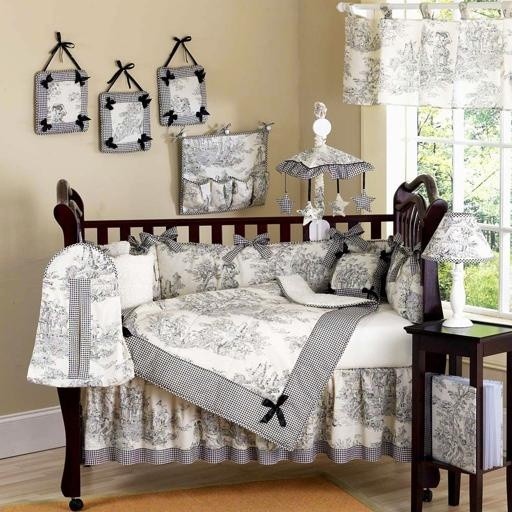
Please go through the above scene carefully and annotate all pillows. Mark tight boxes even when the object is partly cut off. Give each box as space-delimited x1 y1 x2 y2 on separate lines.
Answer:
95 225 424 324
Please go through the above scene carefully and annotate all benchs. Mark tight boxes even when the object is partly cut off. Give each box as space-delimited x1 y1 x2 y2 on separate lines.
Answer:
24 174 448 512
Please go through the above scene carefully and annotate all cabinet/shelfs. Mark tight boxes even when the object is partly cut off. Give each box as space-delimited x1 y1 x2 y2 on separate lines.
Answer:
401 318 510 512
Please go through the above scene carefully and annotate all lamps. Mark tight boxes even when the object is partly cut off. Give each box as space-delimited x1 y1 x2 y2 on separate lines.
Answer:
420 212 494 329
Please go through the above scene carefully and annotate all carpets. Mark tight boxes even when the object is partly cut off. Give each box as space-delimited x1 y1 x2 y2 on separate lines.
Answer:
0 476 371 512
